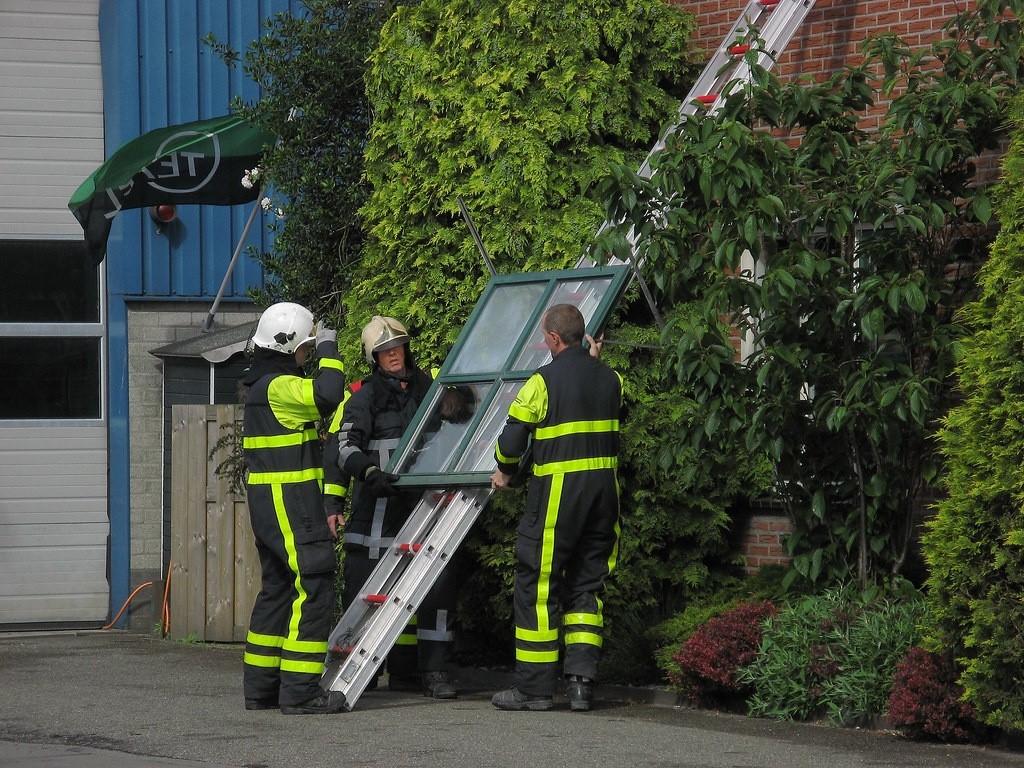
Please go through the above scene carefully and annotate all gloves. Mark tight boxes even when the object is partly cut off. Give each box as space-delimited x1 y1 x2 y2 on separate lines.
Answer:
315 319 337 349
365 469 399 497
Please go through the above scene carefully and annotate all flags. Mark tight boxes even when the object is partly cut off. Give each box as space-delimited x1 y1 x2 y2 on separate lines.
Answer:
67 109 288 274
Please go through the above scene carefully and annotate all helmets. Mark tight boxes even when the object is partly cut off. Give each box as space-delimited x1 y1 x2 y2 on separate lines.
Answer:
252 302 319 355
362 316 411 369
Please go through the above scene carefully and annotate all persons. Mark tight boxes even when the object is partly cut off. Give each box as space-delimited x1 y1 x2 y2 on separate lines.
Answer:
241 302 345 714
490 303 626 710
322 314 466 700
406 385 478 473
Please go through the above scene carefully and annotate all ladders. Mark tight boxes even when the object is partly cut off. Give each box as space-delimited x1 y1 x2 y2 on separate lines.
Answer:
318 0 817 716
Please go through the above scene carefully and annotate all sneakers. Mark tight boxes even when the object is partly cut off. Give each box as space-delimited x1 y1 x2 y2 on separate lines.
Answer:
552 681 593 710
280 687 345 714
492 686 553 710
245 697 279 710
422 676 457 700
